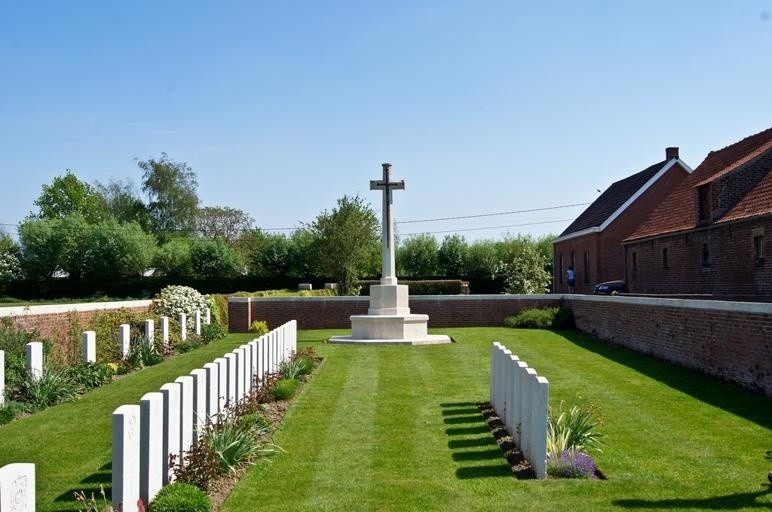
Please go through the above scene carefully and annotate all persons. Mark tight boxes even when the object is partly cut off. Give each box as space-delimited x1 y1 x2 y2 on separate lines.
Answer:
564 265 576 294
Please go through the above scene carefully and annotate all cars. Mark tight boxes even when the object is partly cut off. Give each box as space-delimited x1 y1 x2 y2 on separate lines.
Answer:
594 279 626 296
